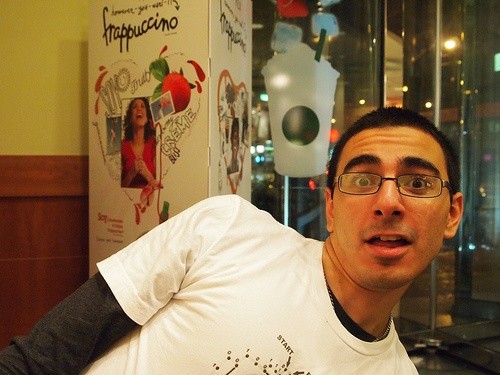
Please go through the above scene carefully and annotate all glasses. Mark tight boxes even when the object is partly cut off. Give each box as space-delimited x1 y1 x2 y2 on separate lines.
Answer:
332 172 454 198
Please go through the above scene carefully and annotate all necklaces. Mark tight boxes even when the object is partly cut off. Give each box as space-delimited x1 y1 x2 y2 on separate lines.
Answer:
323 265 393 342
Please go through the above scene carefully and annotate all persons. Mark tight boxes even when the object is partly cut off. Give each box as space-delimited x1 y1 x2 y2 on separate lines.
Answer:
1 105 464 375
121 97 159 189
